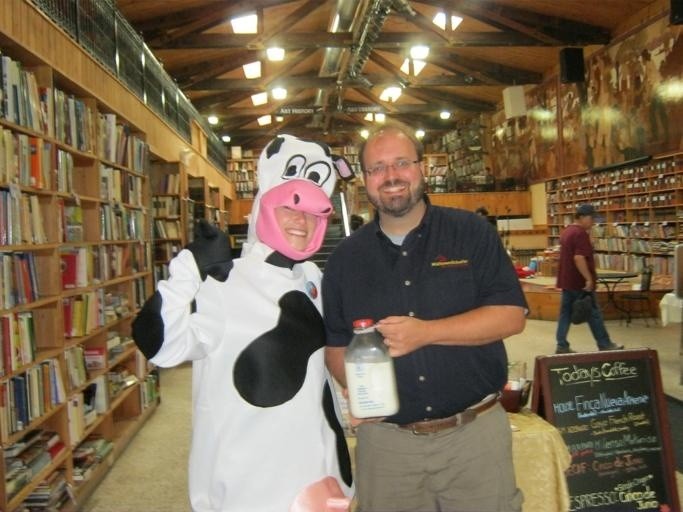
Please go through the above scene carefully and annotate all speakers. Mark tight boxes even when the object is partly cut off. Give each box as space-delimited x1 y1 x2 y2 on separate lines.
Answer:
669 0 682 25
559 46 586 85
502 85 527 120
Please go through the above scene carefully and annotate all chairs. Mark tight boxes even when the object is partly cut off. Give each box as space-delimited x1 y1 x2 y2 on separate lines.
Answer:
620 267 658 328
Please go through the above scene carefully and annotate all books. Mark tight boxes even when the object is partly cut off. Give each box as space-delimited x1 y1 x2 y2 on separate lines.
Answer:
0 123 146 208
0 188 146 246
418 119 496 193
0 428 117 512
0 273 146 379
0 50 146 176
225 145 258 200
1 330 140 446
542 154 683 276
331 144 367 191
0 241 146 317
149 164 181 292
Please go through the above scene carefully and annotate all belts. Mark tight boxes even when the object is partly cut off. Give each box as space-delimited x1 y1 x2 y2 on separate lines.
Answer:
400 396 496 435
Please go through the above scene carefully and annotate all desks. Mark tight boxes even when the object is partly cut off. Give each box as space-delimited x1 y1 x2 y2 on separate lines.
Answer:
595 272 638 327
519 272 676 321
340 407 572 512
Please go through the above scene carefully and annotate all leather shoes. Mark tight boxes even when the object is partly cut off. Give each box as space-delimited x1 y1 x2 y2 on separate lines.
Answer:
598 343 623 350
556 348 578 353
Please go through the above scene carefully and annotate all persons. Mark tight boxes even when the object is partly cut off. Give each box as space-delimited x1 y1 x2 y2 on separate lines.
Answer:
484 166 496 191
557 203 625 355
347 215 365 231
320 119 533 511
475 206 491 218
126 133 357 511
442 162 458 193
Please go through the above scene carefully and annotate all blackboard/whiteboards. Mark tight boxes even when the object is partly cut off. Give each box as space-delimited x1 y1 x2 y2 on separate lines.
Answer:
532 349 681 512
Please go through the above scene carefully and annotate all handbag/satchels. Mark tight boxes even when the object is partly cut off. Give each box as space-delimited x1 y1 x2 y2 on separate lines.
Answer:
571 293 592 324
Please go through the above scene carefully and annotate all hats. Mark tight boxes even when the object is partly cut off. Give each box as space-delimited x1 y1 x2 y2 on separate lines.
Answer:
577 204 599 218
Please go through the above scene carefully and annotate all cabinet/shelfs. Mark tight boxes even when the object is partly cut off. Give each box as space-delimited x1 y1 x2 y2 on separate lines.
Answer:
226 153 452 199
545 152 683 280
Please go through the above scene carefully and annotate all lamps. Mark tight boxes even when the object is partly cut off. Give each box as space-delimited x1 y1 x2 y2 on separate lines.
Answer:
439 111 451 120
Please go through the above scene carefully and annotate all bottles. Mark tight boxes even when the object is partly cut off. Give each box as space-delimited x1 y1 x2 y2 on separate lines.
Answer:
343 317 402 418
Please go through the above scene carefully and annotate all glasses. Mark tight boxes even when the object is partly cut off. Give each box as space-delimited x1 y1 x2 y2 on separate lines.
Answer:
364 160 420 175
